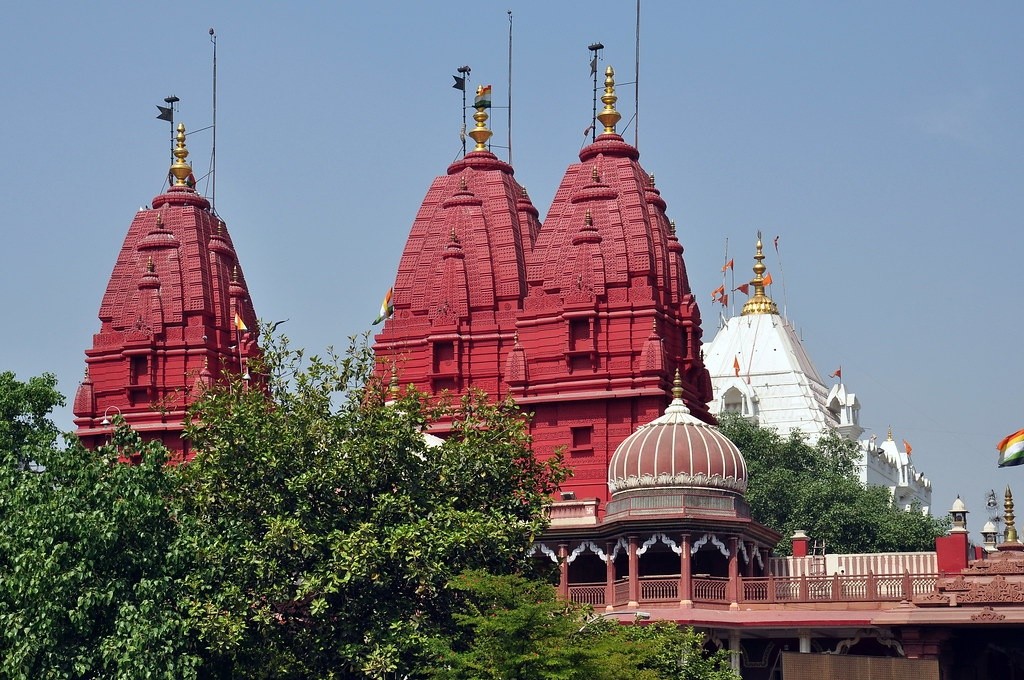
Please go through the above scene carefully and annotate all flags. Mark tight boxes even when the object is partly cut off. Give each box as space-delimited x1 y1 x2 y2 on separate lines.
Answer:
996 430 1024 467
